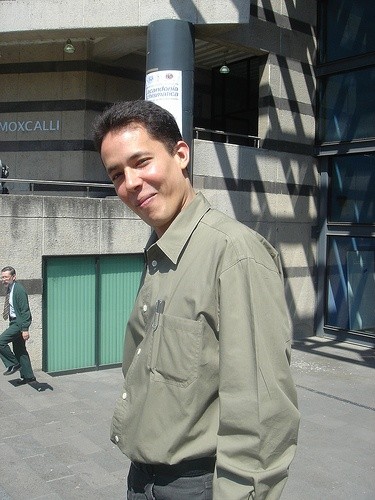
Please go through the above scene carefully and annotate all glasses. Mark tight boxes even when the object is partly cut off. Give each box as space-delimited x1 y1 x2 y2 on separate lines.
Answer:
0 275 12 281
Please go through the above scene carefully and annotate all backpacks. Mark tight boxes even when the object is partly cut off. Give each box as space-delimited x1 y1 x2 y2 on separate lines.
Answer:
1 163 9 178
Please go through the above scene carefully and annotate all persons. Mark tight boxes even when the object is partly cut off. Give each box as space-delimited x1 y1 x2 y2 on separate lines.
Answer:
0 266 37 386
89 100 303 500
0 161 8 193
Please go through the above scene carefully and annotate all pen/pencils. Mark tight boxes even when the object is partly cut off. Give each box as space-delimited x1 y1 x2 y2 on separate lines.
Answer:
152 300 165 330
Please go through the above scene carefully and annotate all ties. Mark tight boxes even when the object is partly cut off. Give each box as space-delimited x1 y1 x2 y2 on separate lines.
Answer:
3 289 10 321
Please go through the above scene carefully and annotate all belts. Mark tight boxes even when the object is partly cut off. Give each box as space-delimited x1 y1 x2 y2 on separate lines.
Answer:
132 457 216 477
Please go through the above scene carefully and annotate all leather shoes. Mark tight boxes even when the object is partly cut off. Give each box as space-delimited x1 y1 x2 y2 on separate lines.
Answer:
3 363 22 375
17 377 36 385
